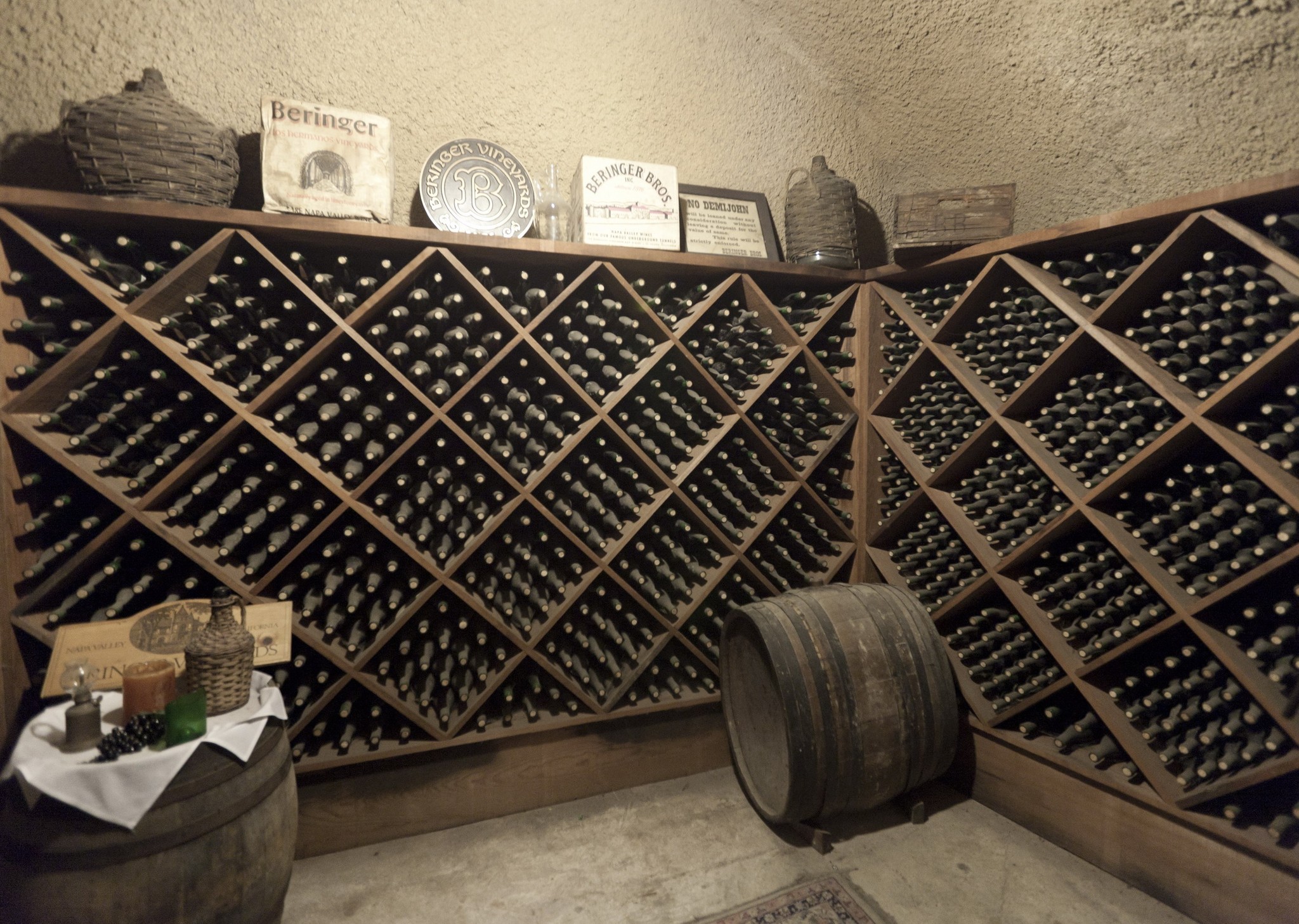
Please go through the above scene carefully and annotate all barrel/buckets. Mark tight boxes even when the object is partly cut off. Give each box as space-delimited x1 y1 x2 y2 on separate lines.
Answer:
720 584 960 823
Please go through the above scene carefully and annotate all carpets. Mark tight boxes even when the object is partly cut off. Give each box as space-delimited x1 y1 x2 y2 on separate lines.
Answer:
682 868 898 924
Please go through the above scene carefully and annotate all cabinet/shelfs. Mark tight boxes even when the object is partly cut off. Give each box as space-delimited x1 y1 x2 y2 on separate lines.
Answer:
0 168 1299 924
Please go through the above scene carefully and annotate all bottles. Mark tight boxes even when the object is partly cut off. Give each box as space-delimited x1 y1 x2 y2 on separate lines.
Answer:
185 587 255 716
277 518 424 660
897 555 1075 710
1053 385 1299 558
785 155 861 270
318 250 421 526
870 281 975 402
1019 691 1299 839
203 254 329 577
878 402 1072 558
656 276 832 339
1061 549 1299 700
12 232 200 625
418 559 662 729
965 213 1299 395
267 654 432 762
410 388 662 565
658 332 858 698
409 256 662 407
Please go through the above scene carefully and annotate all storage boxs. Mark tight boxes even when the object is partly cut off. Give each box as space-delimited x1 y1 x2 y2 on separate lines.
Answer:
570 155 680 250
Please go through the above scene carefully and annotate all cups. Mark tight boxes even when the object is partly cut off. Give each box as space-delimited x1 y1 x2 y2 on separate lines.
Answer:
123 660 177 722
165 686 207 747
